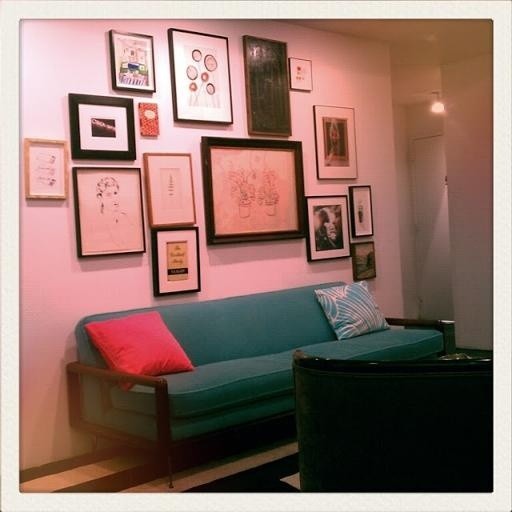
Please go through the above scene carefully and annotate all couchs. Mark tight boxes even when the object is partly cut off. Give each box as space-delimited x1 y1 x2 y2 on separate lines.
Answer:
65 281 456 488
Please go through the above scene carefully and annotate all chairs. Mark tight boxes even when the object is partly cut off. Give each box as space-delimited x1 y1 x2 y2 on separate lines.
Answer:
294 349 492 492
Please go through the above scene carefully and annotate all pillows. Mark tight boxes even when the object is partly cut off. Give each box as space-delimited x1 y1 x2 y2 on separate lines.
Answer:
85 311 195 389
315 280 390 340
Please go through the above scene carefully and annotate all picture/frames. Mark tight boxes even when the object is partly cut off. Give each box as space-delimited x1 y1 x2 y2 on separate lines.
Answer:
289 58 313 92
303 195 351 262
24 138 69 200
110 29 157 92
201 136 305 246
349 185 374 238
72 167 146 257
350 241 376 281
313 105 358 180
243 34 292 137
151 228 200 296
167 28 233 125
143 153 196 227
69 93 138 160
